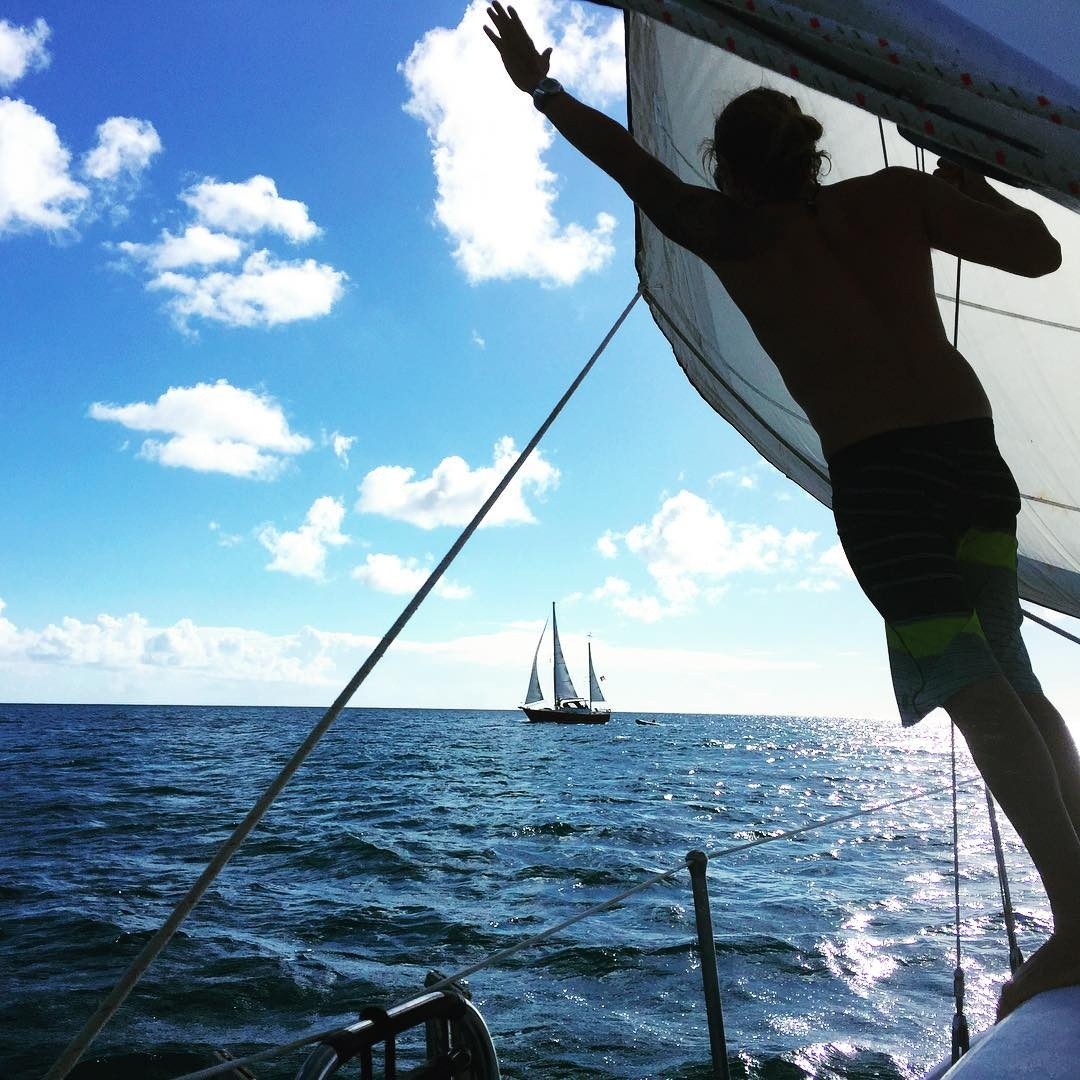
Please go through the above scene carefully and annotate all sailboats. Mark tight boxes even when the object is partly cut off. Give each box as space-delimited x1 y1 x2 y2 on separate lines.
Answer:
518 602 612 725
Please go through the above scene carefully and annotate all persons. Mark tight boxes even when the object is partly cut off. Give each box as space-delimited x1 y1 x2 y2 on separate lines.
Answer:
482 0 1080 1027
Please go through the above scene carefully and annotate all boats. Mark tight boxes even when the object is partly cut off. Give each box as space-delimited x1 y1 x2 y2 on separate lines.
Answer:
635 718 682 728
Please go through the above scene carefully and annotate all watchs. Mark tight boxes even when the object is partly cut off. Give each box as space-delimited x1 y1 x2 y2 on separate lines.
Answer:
533 78 566 113
